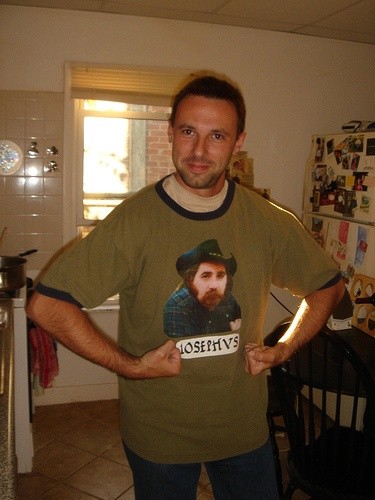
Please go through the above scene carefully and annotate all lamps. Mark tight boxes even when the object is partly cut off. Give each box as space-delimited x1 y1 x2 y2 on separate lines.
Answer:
28 141 38 155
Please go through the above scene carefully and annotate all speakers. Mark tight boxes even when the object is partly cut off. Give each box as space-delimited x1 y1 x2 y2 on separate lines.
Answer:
326 287 354 330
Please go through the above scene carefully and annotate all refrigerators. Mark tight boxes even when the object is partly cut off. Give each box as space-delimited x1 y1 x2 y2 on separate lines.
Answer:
300 132 375 429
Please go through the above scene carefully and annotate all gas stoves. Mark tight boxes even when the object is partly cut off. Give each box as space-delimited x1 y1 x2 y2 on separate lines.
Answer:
0 285 26 306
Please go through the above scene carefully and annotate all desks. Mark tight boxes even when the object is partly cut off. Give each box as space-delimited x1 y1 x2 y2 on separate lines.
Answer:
271 317 375 399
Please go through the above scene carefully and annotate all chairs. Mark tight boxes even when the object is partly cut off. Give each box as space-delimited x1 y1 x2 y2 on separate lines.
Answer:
263 321 375 500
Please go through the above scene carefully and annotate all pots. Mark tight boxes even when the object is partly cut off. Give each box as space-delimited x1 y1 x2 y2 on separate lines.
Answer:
0 249 38 291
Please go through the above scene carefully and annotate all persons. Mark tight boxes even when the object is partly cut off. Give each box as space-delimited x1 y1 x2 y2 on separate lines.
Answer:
160 238 244 339
23 76 347 500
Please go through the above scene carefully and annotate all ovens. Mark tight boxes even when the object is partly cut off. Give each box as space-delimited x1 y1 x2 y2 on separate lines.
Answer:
14 307 34 473
